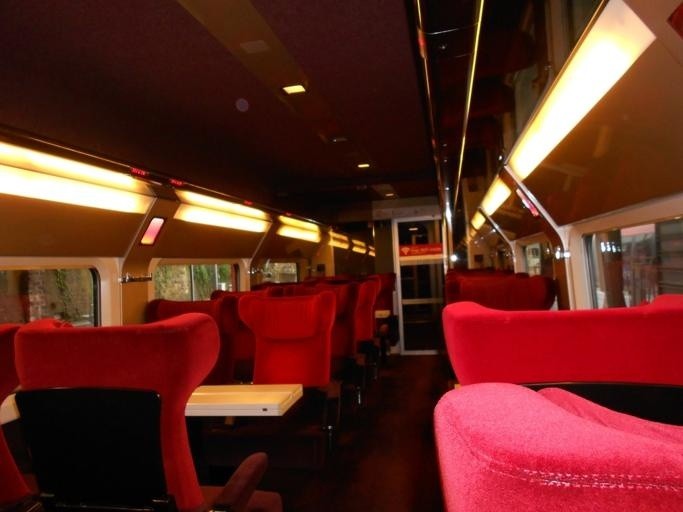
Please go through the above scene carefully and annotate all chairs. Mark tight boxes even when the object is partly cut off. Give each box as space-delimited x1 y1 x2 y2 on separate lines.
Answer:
433 261 683 512
0 262 399 512
